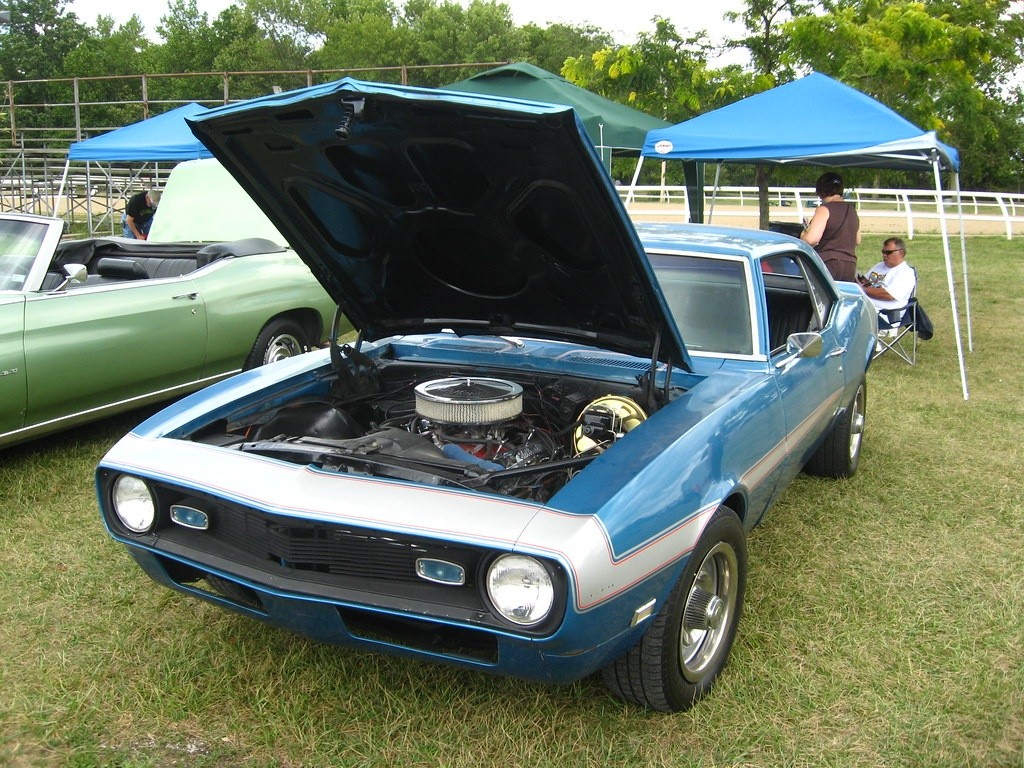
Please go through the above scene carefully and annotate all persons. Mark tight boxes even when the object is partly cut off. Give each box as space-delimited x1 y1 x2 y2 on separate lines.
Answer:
121 189 160 239
855 238 917 351
801 172 861 282
614 172 625 186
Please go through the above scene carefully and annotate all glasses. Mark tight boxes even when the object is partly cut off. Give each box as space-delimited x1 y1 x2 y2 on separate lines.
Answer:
882 248 902 255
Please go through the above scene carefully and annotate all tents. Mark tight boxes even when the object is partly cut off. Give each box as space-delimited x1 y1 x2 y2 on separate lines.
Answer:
54 63 974 401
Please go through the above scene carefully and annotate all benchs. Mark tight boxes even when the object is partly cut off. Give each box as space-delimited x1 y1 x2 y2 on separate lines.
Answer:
657 281 813 350
87 257 196 279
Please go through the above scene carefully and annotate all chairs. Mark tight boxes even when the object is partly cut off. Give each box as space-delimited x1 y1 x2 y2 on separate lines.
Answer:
871 264 919 368
72 259 143 287
40 272 63 290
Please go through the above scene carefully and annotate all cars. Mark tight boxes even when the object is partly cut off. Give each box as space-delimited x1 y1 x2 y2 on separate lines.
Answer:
95 77 877 714
0 158 356 451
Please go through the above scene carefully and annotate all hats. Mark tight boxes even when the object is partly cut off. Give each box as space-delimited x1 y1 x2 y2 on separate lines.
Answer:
146 190 162 209
